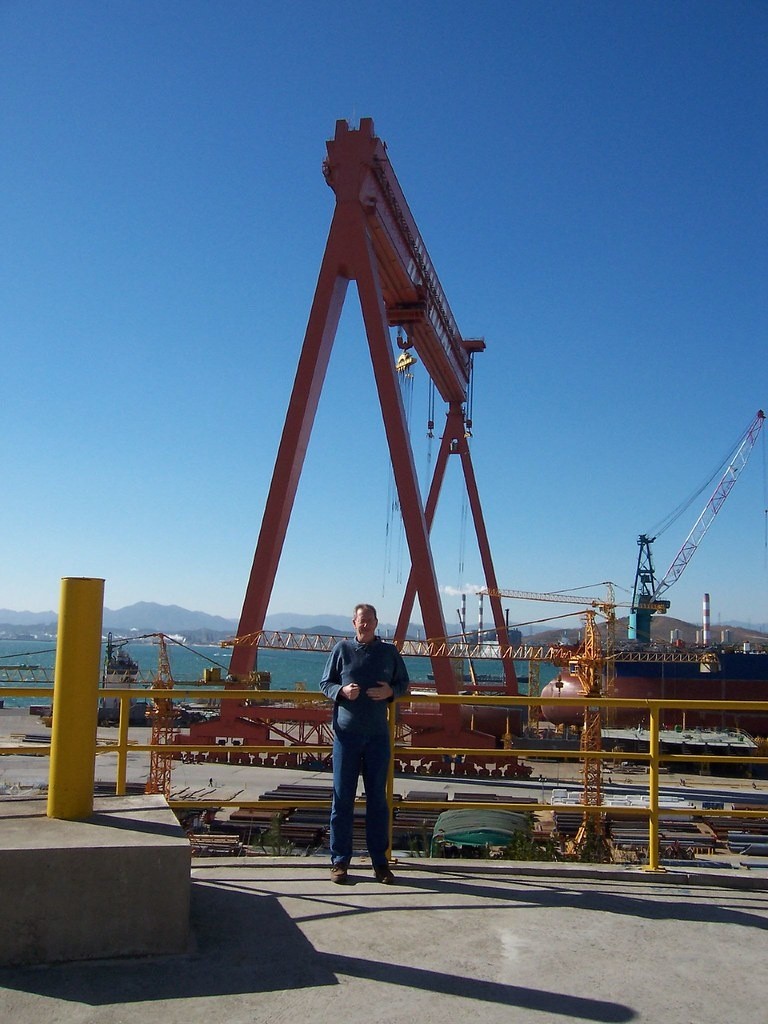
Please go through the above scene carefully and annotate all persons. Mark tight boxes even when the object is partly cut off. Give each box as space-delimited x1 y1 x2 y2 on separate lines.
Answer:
319 604 409 885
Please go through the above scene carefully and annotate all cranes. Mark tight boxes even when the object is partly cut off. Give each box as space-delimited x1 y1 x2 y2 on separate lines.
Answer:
627 408 767 645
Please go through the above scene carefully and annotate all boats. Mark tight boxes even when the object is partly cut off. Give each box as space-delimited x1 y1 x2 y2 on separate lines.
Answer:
104 642 141 684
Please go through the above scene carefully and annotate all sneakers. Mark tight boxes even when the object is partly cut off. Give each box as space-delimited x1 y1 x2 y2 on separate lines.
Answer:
330 863 348 882
373 865 394 883
